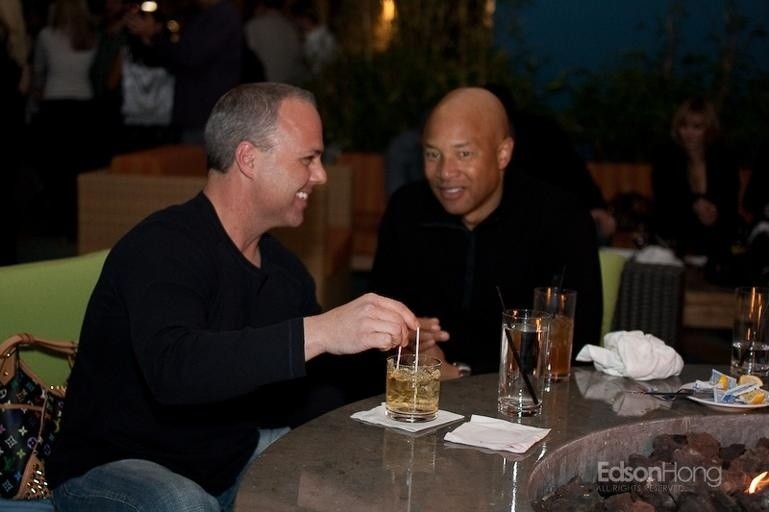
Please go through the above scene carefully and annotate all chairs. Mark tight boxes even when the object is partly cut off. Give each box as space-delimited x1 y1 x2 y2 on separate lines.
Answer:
586 162 754 328
0 249 111 386
76 143 388 312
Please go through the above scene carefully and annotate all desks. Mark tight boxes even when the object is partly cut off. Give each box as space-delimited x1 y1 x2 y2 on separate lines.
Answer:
233 366 769 512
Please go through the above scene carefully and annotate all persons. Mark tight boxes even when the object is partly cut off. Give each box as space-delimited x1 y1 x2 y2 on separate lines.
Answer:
53 83 420 512
366 87 600 382
2 1 191 157
553 99 768 285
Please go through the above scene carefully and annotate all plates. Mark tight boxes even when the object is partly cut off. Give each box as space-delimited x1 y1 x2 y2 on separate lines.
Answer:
686 388 769 412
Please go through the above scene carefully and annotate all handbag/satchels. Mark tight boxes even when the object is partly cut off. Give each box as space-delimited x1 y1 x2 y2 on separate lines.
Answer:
0 333 79 501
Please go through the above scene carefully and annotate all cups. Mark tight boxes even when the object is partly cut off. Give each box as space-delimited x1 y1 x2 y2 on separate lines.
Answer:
385 353 442 424
730 285 769 379
532 287 577 382
382 426 437 474
496 308 551 418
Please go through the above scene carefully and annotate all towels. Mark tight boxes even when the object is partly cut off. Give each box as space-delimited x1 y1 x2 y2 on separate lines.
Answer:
575 330 685 381
